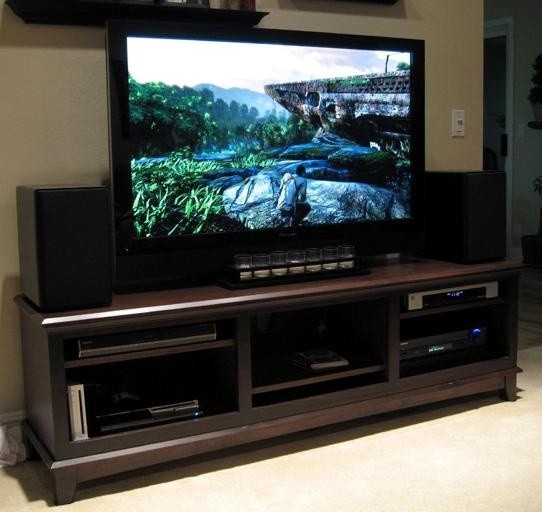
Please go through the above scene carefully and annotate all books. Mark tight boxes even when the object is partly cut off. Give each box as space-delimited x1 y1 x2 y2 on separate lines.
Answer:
292 344 351 370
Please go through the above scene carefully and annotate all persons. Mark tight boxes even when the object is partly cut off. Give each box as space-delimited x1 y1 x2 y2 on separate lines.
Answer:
275 164 311 228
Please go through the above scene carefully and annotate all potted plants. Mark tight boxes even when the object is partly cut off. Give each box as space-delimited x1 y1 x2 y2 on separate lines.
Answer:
527 53 542 121
522 176 542 269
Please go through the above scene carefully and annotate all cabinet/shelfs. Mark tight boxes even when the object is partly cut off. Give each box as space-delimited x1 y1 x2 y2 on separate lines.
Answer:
13 256 525 505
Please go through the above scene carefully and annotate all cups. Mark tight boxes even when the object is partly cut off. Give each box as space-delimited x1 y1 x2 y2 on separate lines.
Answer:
234 245 354 279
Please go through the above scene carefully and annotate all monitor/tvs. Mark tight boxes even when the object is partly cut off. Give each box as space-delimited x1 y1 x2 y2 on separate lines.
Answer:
104 17 426 291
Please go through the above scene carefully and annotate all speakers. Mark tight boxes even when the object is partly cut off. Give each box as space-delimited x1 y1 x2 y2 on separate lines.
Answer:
399 168 508 263
16 182 113 310
